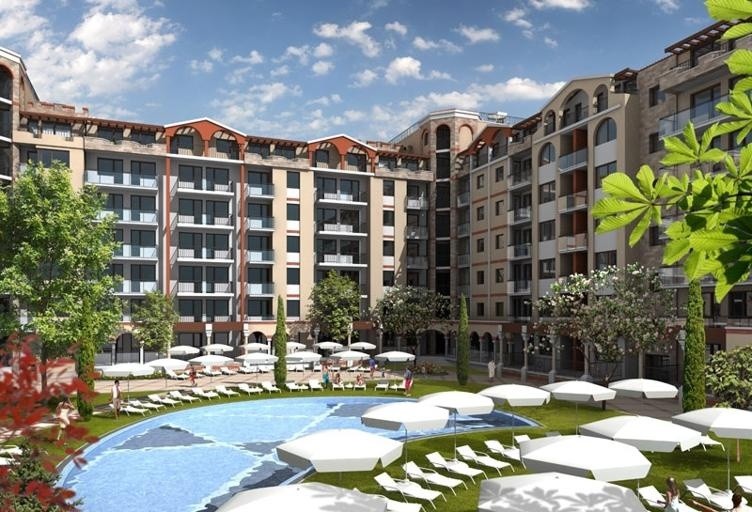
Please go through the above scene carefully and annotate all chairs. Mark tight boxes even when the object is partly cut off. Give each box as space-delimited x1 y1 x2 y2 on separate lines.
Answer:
108 379 413 418
349 429 566 512
0 442 26 457
679 476 739 511
0 458 23 468
731 474 751 495
635 484 699 511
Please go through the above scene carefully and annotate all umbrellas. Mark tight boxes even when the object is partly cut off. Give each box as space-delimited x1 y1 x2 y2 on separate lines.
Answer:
476 470 650 512
215 481 388 511
672 406 752 490
610 376 680 414
416 390 495 460
361 401 449 481
578 414 702 498
538 380 617 434
476 383 551 448
519 433 653 482
276 427 404 481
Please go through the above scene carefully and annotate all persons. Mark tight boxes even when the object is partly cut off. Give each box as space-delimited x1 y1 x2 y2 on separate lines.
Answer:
403 366 412 397
421 365 427 379
108 379 121 421
55 396 75 441
657 477 681 512
487 359 496 381
728 492 745 512
